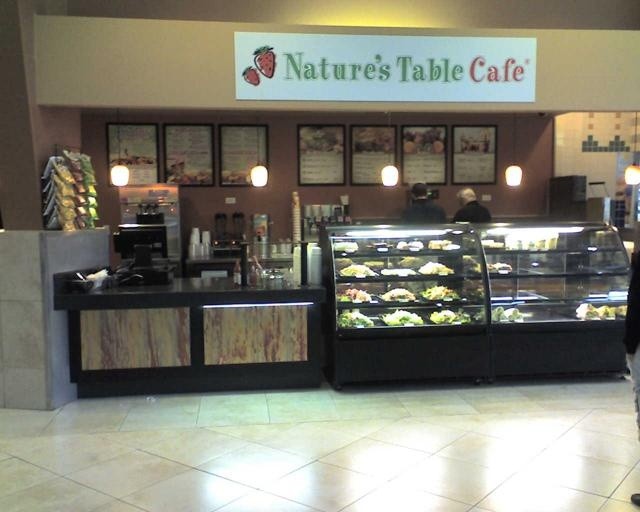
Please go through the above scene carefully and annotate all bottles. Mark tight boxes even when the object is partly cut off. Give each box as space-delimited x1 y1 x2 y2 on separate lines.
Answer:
271 238 292 254
249 261 257 287
107 276 113 288
232 260 241 287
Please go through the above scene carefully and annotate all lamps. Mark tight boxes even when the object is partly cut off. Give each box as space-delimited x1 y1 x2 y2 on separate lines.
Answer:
247 113 268 189
380 112 401 189
503 115 523 187
109 108 130 189
626 111 640 187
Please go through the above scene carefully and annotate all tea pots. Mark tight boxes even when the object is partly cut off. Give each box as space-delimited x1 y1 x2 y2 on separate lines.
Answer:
259 269 284 290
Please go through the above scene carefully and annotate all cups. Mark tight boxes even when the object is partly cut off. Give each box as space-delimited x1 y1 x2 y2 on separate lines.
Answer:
290 192 323 286
188 226 211 259
321 205 352 226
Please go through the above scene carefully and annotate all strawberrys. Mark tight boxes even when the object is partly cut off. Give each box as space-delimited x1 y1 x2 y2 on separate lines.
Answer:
254 45 275 78
241 66 259 86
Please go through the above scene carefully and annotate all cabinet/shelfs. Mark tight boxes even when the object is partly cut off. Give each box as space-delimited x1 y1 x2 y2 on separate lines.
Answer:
322 215 489 392
479 222 631 384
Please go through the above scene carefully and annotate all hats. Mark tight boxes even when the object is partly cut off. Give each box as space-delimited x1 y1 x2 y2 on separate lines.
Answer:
411 184 425 193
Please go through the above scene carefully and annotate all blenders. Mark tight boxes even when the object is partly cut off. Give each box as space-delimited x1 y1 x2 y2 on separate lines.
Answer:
213 212 247 252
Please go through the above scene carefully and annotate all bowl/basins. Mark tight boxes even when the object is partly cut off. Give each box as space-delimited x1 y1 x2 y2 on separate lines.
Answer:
65 280 94 295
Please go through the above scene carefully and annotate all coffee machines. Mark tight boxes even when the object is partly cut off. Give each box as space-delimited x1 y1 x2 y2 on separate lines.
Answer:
113 203 180 283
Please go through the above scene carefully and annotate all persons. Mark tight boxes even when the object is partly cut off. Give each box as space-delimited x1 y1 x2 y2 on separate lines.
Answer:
452 187 492 221
400 182 448 223
621 260 640 506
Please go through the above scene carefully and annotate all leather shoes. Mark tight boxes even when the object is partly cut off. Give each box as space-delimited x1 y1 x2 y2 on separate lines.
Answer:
631 494 640 506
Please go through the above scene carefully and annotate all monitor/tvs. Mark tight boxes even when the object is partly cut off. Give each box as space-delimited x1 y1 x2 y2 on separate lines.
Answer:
112 223 168 270
